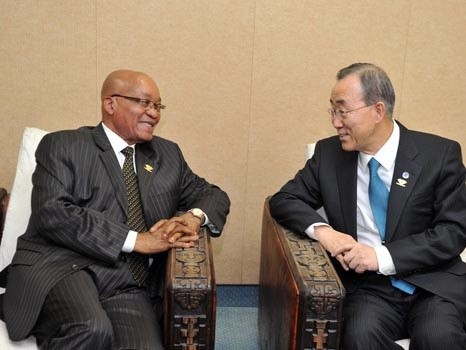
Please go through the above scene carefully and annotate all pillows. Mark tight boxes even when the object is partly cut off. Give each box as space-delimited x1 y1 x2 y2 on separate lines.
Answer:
305 143 330 227
1 125 51 273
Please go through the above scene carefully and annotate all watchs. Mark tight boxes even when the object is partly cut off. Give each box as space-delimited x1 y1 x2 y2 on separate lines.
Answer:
187 206 206 225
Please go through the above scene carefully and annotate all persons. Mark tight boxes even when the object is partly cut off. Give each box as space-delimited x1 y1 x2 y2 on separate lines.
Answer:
1 69 232 350
271 62 466 350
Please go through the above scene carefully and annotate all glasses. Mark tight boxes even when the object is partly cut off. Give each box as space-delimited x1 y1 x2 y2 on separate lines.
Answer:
328 101 377 122
111 94 166 112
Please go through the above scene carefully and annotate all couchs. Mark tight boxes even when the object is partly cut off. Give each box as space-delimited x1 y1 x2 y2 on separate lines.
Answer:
0 186 217 349
256 192 347 350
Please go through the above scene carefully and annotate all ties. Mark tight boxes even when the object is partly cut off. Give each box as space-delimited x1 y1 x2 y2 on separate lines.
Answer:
121 146 151 286
367 157 416 294
